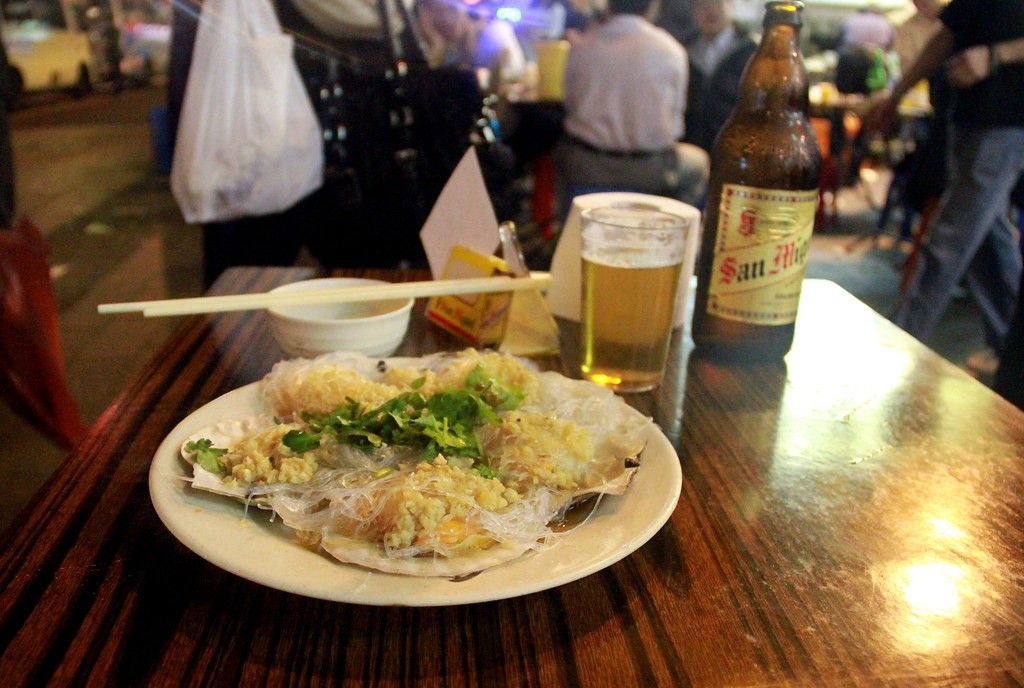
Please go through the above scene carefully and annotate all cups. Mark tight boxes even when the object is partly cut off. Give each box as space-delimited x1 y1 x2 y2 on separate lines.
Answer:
580 206 690 394
533 41 569 98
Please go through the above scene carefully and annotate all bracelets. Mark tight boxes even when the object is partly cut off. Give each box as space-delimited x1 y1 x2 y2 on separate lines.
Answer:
987 44 1002 76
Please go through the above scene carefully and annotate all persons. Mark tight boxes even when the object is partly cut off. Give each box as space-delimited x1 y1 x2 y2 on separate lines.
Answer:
197 0 1022 409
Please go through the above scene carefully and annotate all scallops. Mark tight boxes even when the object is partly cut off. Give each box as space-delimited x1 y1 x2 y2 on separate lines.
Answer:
181 347 649 577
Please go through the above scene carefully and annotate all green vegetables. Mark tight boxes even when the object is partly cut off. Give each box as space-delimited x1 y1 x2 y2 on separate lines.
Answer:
185 360 526 483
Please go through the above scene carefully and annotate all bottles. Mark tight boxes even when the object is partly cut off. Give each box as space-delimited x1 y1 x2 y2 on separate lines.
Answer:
692 1 824 358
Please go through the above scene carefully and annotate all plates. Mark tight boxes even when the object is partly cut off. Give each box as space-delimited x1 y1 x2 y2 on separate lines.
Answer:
149 360 682 606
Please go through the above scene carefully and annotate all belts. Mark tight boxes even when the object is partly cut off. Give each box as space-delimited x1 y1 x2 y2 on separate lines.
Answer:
564 132 674 158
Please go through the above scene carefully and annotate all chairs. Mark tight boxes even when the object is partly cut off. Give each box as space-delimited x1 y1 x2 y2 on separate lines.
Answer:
811 156 1022 304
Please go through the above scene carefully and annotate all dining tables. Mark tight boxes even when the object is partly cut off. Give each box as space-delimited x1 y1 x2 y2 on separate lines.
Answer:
807 86 933 153
1 265 1024 688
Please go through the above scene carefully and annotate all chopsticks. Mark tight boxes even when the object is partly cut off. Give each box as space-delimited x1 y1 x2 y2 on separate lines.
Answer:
97 276 556 319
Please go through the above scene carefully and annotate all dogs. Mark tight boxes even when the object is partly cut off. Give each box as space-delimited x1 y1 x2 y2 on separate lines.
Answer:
869 111 952 255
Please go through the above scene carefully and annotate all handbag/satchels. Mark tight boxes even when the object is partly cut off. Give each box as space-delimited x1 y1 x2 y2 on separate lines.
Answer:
155 1 482 269
170 1 326 223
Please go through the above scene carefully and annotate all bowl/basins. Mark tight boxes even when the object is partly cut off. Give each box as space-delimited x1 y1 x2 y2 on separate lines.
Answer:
266 278 415 359
